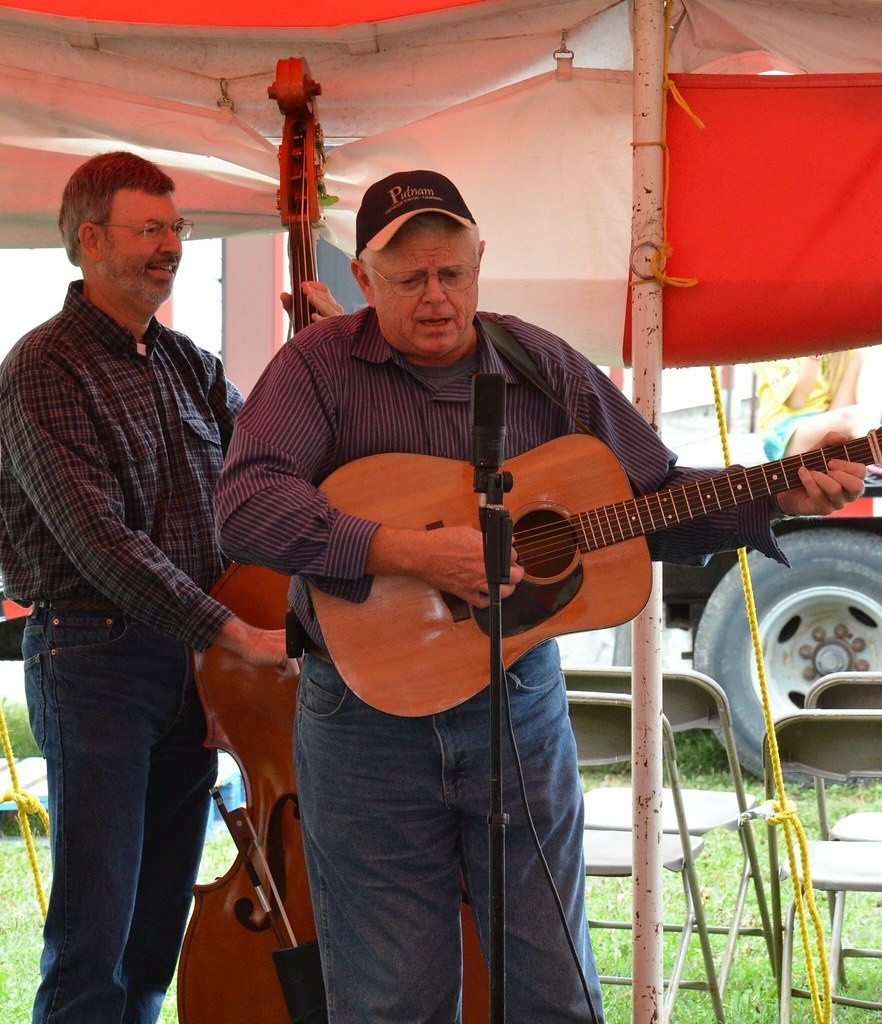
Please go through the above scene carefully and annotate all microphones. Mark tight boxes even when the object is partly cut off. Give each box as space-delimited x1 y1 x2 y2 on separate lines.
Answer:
471 374 507 532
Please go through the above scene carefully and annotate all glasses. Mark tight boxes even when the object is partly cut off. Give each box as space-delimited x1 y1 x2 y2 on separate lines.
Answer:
79 220 194 243
361 260 479 298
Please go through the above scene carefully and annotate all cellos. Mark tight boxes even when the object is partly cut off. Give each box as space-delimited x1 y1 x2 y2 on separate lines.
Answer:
114 55 489 1024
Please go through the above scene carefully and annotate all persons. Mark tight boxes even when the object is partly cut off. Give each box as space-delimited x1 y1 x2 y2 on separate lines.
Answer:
214 169 866 1024
753 347 882 498
0 149 343 1024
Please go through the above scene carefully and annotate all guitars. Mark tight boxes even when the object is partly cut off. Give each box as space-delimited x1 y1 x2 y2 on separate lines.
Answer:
303 424 882 719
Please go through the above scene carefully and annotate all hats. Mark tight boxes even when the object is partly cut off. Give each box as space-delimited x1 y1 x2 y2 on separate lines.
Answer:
356 170 477 257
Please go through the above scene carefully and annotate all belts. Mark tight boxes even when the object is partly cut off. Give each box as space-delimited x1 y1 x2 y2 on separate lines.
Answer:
31 595 130 613
302 630 333 665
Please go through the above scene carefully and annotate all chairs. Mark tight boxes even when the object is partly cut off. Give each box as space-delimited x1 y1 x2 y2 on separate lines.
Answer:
563 665 882 1024
